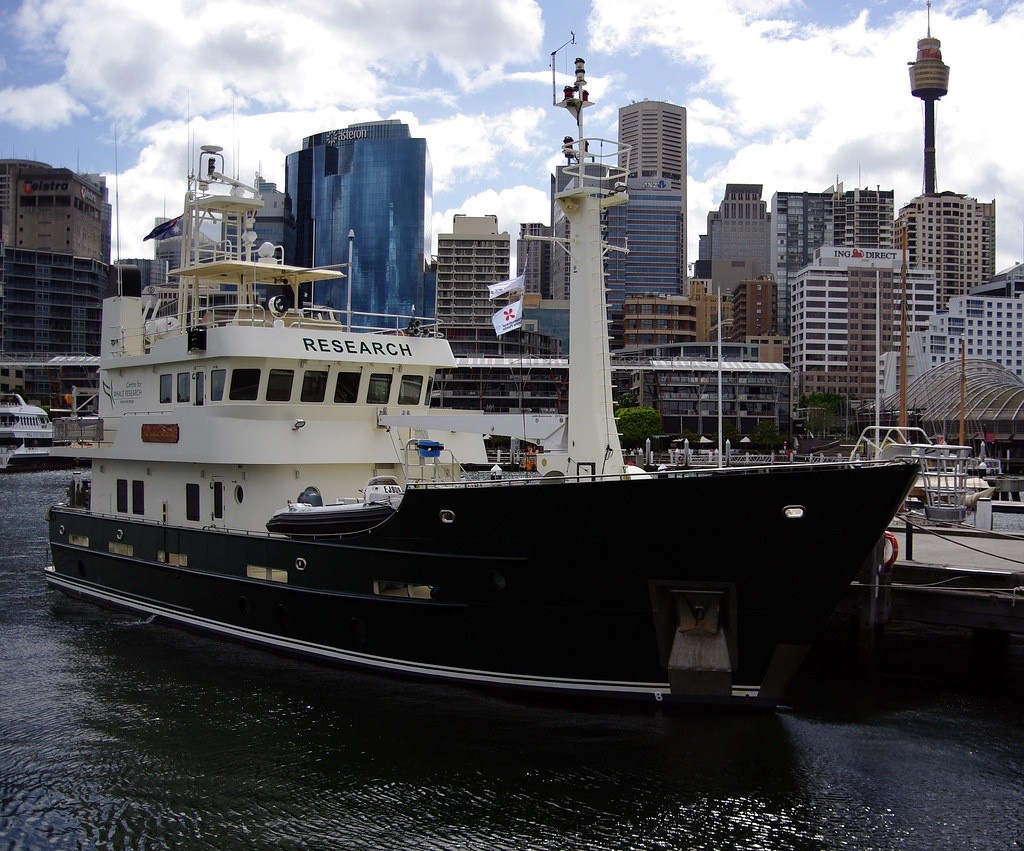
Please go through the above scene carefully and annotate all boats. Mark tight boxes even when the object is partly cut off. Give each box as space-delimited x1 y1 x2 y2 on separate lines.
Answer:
0 391 57 473
43 26 929 705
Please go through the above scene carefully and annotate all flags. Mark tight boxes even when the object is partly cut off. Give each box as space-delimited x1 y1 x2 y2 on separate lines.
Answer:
487 257 525 297
143 212 189 239
492 298 522 336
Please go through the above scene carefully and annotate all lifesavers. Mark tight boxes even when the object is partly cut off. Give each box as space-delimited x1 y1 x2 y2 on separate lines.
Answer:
880 531 899 567
